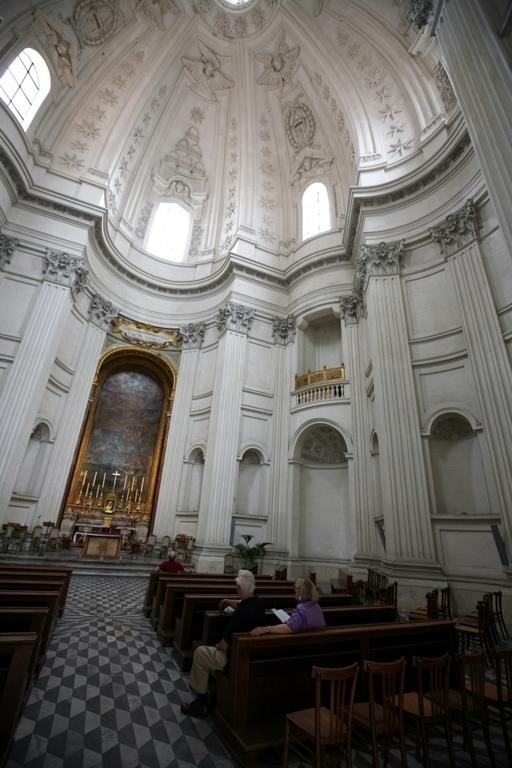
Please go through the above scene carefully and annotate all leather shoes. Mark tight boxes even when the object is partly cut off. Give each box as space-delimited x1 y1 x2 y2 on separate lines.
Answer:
180 701 206 718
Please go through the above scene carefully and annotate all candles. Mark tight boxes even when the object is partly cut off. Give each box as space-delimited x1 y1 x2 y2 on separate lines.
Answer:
78 469 144 514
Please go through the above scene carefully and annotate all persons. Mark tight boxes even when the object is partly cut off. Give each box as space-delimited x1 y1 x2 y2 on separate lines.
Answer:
180 568 266 719
249 577 325 638
153 550 192 574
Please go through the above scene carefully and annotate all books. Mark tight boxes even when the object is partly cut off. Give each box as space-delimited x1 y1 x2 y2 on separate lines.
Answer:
271 606 291 624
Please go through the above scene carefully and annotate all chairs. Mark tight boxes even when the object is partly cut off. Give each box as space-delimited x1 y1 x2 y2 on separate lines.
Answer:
337 657 407 767
0 523 196 563
425 591 511 767
382 655 456 767
284 663 359 767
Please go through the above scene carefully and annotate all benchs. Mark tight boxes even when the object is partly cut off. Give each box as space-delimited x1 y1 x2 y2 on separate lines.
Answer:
142 568 458 768
0 560 74 768
